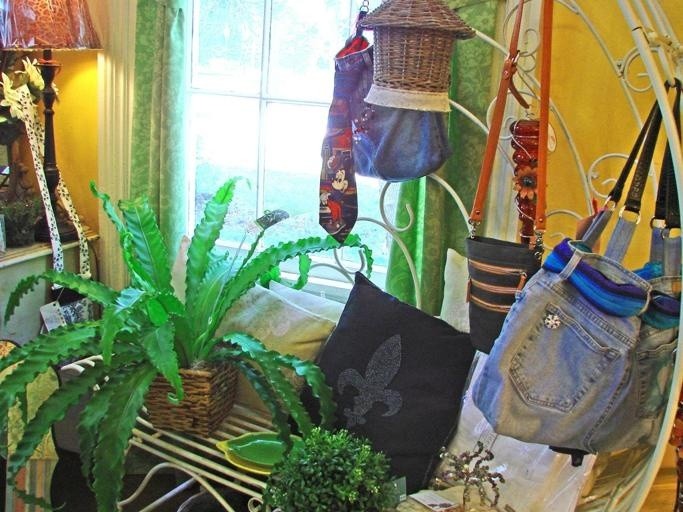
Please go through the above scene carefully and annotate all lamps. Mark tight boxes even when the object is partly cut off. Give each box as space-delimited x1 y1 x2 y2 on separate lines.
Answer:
0 0 103 244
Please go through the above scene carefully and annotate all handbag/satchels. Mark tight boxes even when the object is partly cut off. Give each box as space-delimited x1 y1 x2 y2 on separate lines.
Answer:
319 0 454 182
39 274 94 339
466 237 542 355
473 239 682 454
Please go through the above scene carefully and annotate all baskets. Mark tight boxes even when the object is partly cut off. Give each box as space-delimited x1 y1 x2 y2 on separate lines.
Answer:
144 361 238 436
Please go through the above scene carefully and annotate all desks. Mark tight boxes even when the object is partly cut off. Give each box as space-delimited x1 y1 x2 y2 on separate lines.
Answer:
49 354 291 512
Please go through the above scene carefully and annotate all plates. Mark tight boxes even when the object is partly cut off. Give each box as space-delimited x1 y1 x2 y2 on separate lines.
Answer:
216 431 301 475
226 433 297 467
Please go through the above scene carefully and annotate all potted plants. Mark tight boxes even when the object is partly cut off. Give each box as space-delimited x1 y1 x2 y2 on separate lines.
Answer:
0 178 374 512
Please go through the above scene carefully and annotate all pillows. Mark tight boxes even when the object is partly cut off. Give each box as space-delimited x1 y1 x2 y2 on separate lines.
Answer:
281 272 479 494
428 351 603 512
209 282 335 420
267 279 345 326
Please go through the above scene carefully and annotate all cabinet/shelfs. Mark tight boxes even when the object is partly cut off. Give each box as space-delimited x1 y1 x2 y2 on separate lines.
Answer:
0 231 98 349
